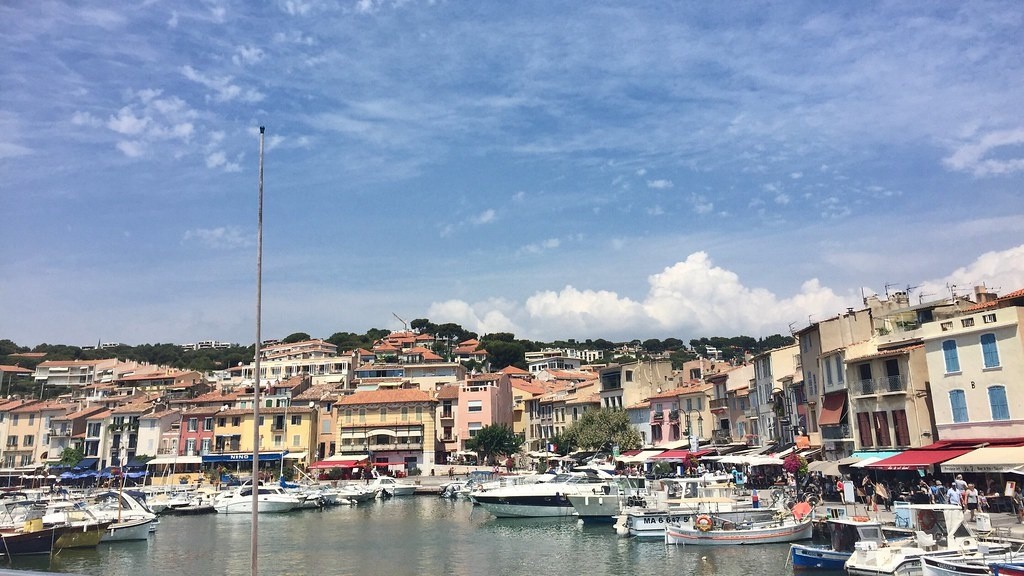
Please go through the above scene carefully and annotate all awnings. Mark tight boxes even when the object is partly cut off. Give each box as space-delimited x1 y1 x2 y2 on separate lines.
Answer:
863 442 989 470
49 455 100 469
202 450 309 462
123 456 156 467
939 446 1024 476
96 466 153 478
307 454 408 469
146 456 202 464
622 449 717 463
849 451 902 459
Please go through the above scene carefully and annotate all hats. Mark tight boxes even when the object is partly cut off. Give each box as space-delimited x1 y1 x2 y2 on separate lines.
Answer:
952 482 956 486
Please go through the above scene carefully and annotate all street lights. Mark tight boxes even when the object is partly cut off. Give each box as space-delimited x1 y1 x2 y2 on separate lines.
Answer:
670 408 703 478
766 387 809 505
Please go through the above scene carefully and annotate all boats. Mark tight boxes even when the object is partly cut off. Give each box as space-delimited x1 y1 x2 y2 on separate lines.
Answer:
0 449 417 576
437 428 1024 576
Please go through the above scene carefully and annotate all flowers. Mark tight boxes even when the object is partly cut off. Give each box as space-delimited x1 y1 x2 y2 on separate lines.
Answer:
784 452 809 479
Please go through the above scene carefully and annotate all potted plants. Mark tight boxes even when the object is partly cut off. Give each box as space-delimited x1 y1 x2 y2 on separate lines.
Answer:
411 467 423 485
207 468 219 487
319 469 327 480
328 466 344 487
178 474 191 484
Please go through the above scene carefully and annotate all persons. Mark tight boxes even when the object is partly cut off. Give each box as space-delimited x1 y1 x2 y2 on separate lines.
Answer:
809 471 998 523
696 462 711 478
567 464 571 473
715 466 738 483
446 455 460 465
466 467 469 477
361 469 365 482
787 472 796 498
743 469 766 490
449 467 456 480
615 464 644 476
492 465 499 474
506 457 516 473
1012 482 1024 524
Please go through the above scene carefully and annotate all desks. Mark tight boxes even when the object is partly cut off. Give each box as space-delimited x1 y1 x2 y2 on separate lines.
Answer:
985 495 1009 513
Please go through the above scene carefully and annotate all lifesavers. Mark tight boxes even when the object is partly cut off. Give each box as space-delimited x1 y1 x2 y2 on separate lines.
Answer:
918 510 938 530
696 515 712 532
853 516 869 522
478 485 483 490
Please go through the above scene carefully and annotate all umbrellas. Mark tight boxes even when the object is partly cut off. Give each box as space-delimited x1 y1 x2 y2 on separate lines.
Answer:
56 469 99 489
717 455 785 468
805 456 883 483
524 450 578 473
18 474 57 488
459 450 478 456
614 453 645 469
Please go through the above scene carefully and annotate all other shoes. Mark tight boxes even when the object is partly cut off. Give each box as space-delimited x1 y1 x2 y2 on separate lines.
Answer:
867 507 869 511
971 518 976 521
